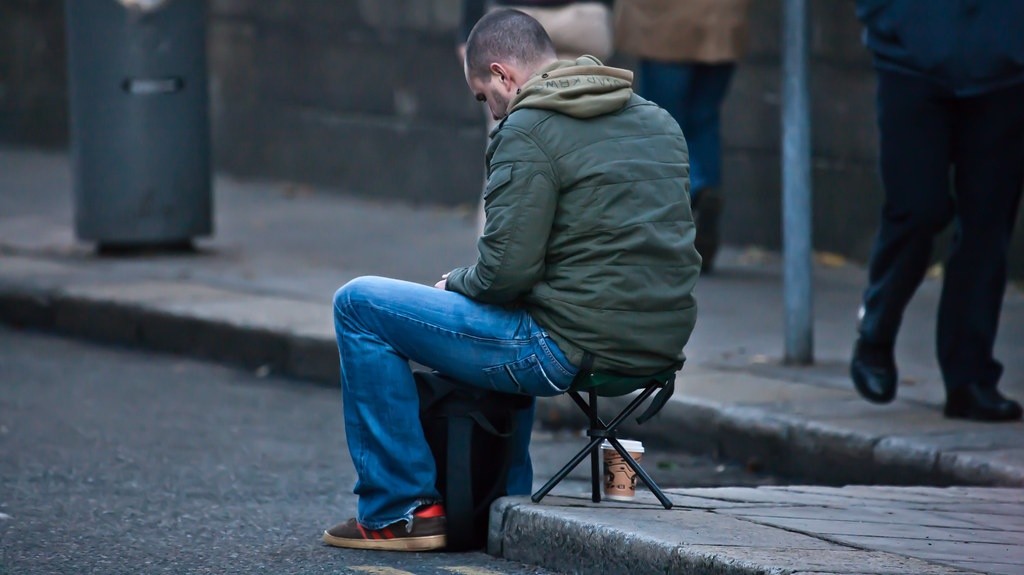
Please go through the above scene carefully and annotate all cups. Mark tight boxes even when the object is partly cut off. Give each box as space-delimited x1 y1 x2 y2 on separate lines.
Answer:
600 437 645 501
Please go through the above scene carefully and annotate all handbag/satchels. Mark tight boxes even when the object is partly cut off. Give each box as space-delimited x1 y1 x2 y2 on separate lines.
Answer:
412 369 515 554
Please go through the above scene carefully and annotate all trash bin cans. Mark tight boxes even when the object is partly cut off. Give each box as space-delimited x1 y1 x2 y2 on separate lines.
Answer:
64 0 216 259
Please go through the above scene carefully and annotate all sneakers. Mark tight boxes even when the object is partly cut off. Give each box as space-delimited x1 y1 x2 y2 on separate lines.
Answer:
320 503 447 552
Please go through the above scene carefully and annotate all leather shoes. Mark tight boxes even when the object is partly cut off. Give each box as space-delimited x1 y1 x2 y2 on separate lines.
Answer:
944 375 1023 423
850 337 897 404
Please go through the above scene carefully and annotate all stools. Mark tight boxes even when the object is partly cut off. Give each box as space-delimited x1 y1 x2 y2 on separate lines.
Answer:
532 350 685 513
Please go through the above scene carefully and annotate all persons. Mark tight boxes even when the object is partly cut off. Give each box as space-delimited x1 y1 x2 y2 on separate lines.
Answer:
321 8 703 552
614 1 745 277
851 1 1024 425
